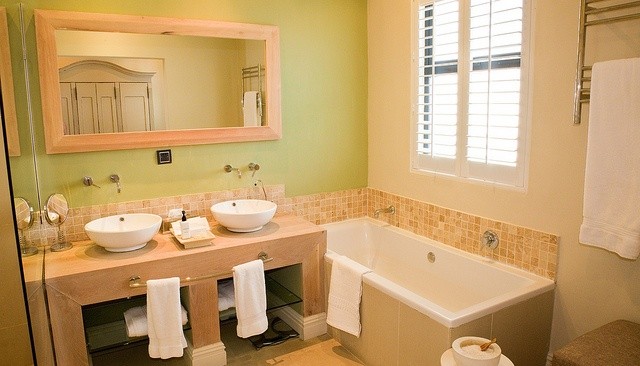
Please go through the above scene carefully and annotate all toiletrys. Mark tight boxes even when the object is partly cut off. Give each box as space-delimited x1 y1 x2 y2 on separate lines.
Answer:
180 211 191 239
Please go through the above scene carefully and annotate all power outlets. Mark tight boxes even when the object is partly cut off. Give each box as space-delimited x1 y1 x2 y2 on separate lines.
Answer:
156 149 173 165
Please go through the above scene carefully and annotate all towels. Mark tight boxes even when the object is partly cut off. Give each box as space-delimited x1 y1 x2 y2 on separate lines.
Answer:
232 258 269 339
240 90 263 126
325 254 374 339
121 302 190 339
217 280 235 313
145 276 188 360
170 216 211 237
576 56 640 262
48 211 58 221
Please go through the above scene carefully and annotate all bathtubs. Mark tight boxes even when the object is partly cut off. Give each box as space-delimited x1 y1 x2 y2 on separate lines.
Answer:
317 216 556 328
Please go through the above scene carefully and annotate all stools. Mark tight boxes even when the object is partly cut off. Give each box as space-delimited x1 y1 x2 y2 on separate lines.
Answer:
550 318 640 365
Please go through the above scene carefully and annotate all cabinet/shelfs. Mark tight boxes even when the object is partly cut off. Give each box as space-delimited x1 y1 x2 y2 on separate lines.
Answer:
82 294 192 355
219 274 304 324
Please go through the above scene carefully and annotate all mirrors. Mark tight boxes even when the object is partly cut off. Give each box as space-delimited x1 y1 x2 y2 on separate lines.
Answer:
43 192 73 253
0 0 56 366
32 7 284 157
0 7 23 159
13 196 39 258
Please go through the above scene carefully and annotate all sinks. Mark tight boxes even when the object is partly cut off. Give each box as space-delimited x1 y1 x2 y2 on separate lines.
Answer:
209 198 277 233
82 213 163 252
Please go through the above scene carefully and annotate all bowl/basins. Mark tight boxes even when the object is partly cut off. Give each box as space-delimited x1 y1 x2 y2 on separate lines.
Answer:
451 336 501 366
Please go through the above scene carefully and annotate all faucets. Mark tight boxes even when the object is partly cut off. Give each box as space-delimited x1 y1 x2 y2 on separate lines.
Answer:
222 164 244 180
374 205 395 220
108 174 121 194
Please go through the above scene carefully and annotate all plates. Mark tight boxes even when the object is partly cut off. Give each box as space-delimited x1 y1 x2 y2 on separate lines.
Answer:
440 347 513 366
